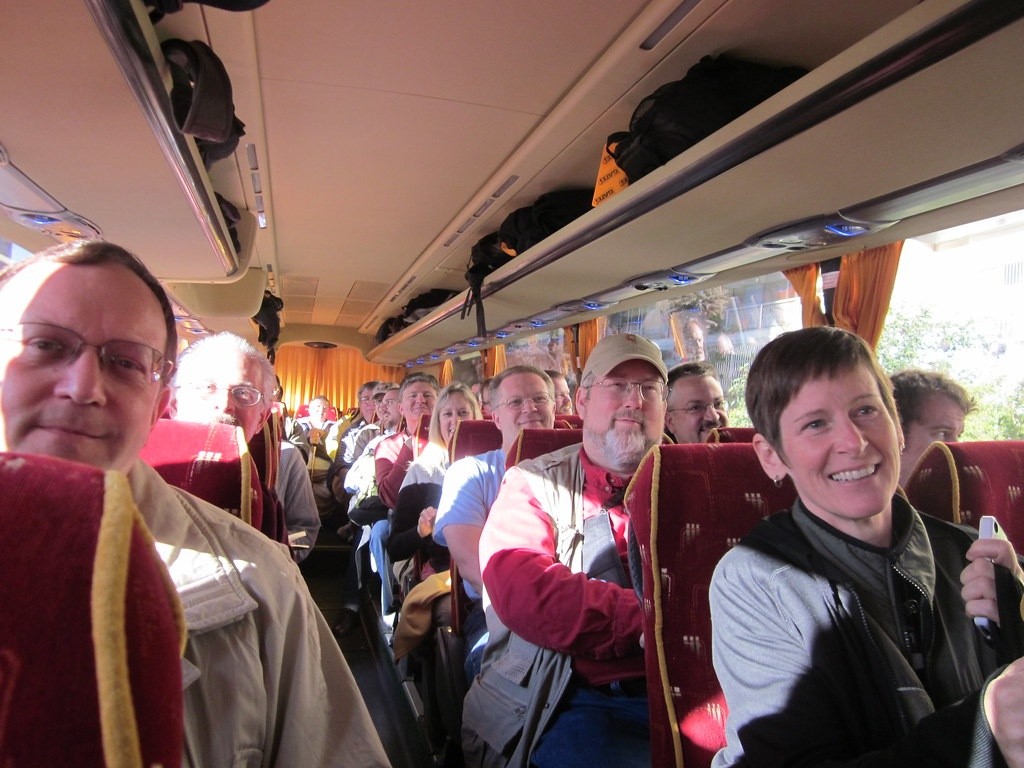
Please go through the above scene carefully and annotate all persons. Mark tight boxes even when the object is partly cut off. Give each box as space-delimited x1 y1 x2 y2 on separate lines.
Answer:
710 324 1023 768
663 317 734 365
166 330 293 567
883 372 978 507
1 235 392 768
478 332 706 768
275 437 323 564
353 365 572 768
295 387 338 446
662 361 732 445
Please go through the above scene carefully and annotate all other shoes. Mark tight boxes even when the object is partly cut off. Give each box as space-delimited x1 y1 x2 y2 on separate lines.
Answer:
337 524 353 543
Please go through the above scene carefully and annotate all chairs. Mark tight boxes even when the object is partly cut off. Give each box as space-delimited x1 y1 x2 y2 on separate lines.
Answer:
0 405 1024 768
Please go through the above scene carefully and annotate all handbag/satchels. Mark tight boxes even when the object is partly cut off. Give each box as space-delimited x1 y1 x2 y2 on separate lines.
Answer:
593 53 808 205
400 289 460 324
374 314 409 343
464 190 588 285
346 457 388 526
253 290 283 365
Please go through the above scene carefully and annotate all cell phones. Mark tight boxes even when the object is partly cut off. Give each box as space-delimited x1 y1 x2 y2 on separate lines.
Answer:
975 516 1008 639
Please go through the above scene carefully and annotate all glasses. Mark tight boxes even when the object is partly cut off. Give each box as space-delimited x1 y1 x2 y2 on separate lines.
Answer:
0 322 173 385
669 401 729 415
584 377 671 404
495 392 554 410
360 397 371 404
176 380 268 408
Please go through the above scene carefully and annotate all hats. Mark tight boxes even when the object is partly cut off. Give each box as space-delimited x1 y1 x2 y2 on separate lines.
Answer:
371 381 400 401
580 333 668 384
